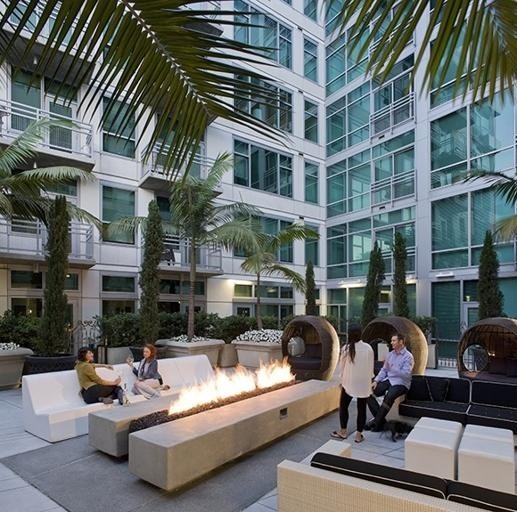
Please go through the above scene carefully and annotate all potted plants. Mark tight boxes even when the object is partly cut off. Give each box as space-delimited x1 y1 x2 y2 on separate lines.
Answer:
416 316 438 368
24 189 225 375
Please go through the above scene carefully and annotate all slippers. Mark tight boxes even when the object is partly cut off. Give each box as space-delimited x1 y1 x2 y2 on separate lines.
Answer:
355 435 364 442
330 431 347 439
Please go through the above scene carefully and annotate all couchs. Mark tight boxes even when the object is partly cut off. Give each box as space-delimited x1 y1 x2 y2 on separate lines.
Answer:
21 355 217 443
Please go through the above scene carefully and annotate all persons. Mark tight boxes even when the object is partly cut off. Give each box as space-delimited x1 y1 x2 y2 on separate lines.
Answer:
326 327 375 443
74 347 131 407
360 332 414 434
125 343 170 400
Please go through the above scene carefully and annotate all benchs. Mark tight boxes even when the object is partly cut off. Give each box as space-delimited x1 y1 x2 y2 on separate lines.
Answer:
399 374 516 435
277 453 517 512
88 376 342 493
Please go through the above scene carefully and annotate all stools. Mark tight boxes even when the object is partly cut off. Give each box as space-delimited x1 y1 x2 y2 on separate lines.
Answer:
458 424 515 495
402 417 461 479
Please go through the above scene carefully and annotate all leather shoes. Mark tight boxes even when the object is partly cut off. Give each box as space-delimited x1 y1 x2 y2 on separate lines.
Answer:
99 397 113 404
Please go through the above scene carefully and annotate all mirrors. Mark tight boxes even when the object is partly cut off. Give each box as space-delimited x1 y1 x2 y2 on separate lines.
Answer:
463 344 488 371
370 337 389 362
287 337 305 357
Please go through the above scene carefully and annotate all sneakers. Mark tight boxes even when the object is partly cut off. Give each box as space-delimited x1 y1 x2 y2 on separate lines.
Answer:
365 418 388 432
161 385 170 389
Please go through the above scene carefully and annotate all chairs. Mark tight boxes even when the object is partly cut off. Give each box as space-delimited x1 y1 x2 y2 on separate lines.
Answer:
362 317 428 375
456 317 517 384
284 318 340 381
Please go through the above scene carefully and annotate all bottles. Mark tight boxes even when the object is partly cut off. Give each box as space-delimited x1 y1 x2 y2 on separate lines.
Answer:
121 382 130 407
